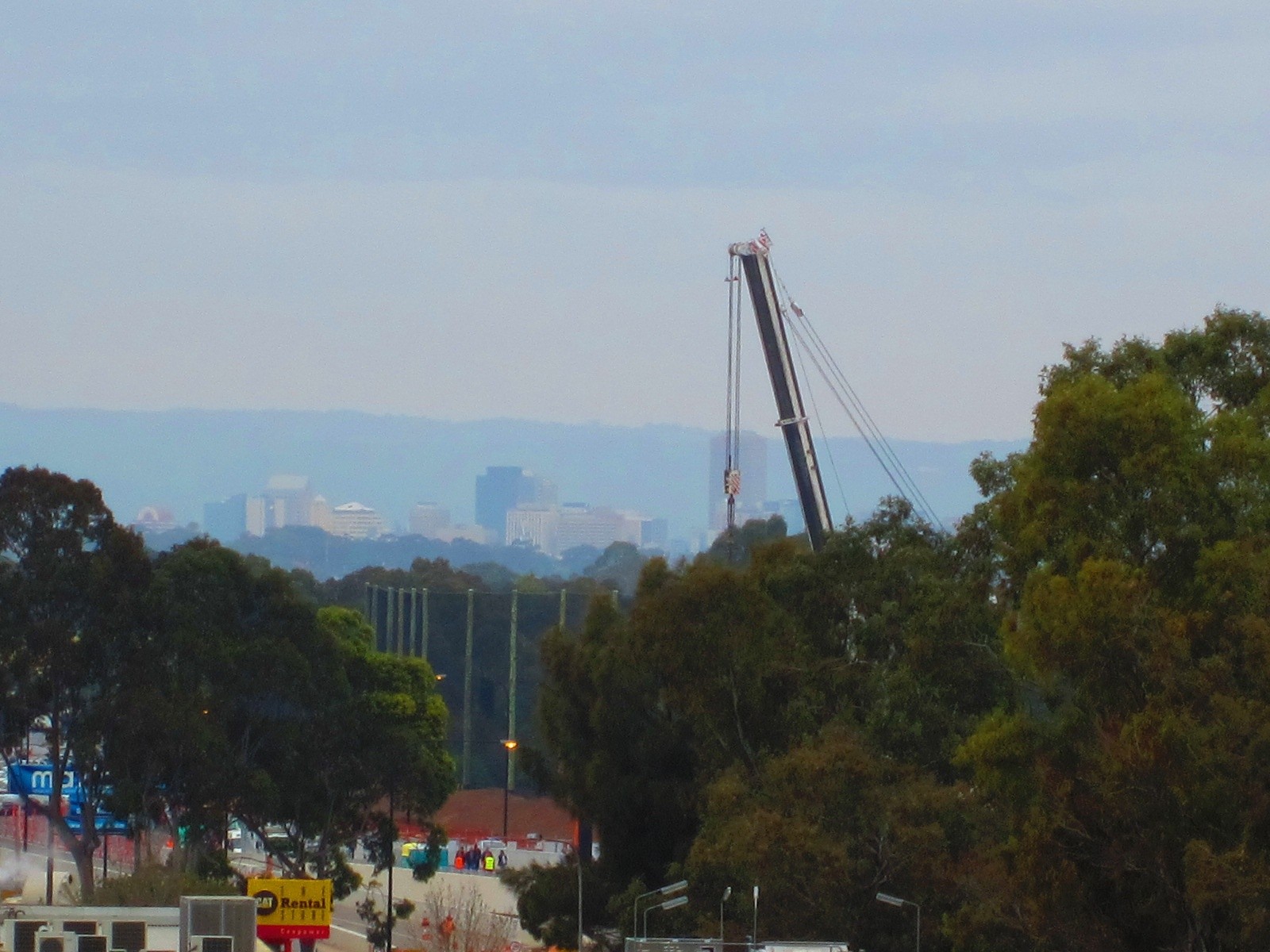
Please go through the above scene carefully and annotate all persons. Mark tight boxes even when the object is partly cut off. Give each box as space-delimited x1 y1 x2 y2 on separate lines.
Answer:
455 843 508 872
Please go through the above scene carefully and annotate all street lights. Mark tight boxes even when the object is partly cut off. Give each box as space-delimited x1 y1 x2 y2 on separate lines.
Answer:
503 738 517 847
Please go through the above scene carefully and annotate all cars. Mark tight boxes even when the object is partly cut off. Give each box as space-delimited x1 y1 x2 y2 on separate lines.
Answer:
0 802 16 815
160 834 173 868
0 771 7 782
446 840 463 849
477 837 507 850
